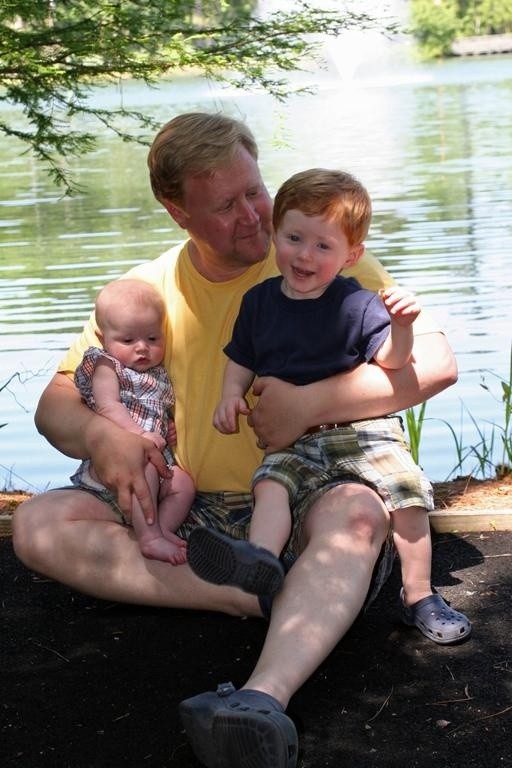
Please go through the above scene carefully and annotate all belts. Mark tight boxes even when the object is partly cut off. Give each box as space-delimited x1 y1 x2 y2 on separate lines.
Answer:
304 421 350 435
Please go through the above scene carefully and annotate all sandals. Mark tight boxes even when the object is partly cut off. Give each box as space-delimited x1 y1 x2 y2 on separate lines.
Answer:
399 584 473 647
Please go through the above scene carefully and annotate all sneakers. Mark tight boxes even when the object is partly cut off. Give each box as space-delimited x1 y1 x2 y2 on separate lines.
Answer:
178 681 300 768
185 526 285 598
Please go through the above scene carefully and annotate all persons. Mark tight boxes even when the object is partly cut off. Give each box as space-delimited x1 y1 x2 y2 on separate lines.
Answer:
8 112 391 768
185 167 474 644
72 278 197 566
